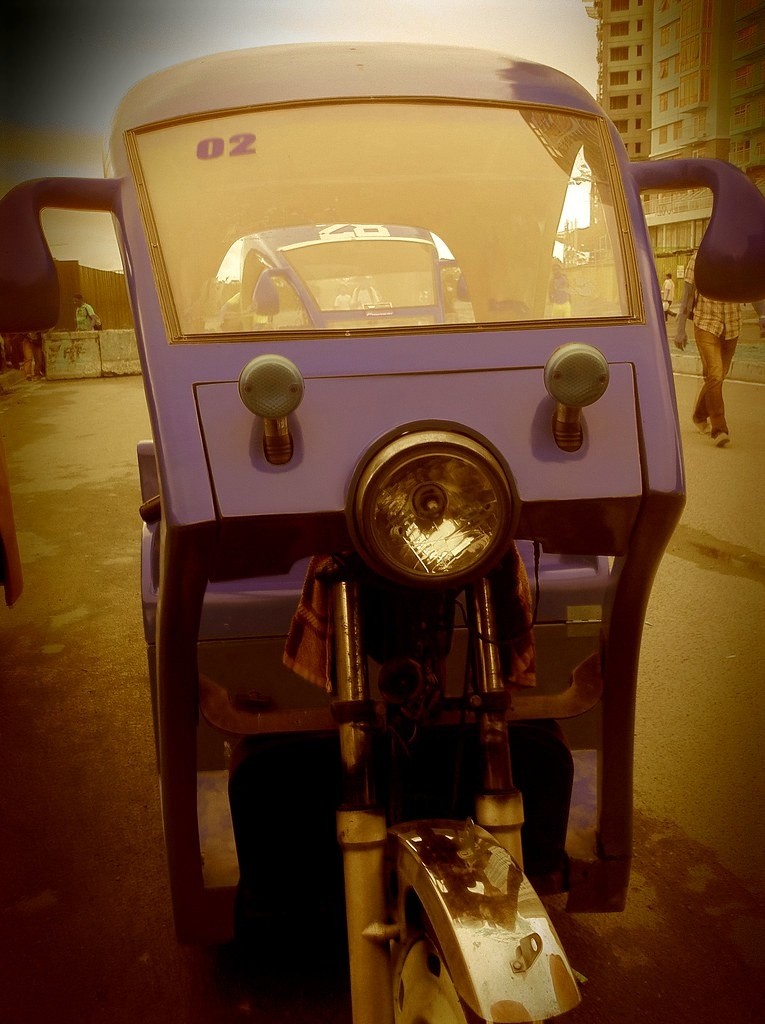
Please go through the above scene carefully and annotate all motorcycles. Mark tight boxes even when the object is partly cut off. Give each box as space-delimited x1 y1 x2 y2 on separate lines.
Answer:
1 43 764 1023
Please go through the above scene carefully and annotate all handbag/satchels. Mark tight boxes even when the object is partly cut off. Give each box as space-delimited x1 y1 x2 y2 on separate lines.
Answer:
663 300 669 311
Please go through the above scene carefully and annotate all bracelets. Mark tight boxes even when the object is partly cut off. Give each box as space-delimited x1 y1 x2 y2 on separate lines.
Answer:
758 315 765 318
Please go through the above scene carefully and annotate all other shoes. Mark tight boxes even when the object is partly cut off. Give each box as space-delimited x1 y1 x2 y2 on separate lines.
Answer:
675 313 678 322
665 321 668 323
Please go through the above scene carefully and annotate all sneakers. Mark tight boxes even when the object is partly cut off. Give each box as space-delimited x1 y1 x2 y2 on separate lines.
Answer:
712 429 729 444
26 374 33 380
693 412 710 435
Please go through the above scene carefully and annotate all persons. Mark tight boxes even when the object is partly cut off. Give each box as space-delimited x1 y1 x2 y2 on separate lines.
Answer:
455 273 473 303
674 243 765 447
0 332 46 381
547 264 572 318
74 293 101 332
661 273 677 324
332 275 379 310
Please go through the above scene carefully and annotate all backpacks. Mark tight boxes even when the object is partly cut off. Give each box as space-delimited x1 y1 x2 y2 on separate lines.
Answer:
93 312 102 330
